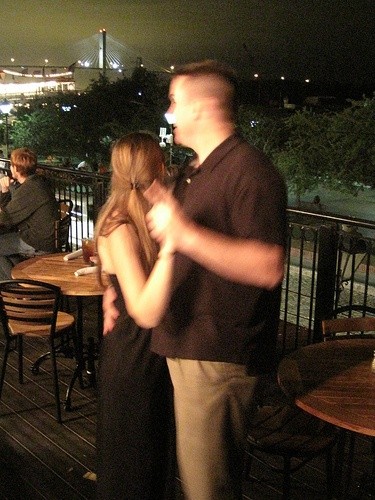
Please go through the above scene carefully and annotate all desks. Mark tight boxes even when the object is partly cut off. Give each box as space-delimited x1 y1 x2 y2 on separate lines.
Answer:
276 337 375 500
11 253 138 412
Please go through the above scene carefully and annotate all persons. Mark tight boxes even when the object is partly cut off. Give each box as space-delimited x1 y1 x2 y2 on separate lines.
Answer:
100 60 287 500
0 147 56 280
94 131 176 500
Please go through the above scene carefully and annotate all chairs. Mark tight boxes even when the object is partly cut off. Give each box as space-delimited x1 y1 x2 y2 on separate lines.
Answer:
51 200 73 253
244 337 344 500
0 279 84 423
322 305 375 341
